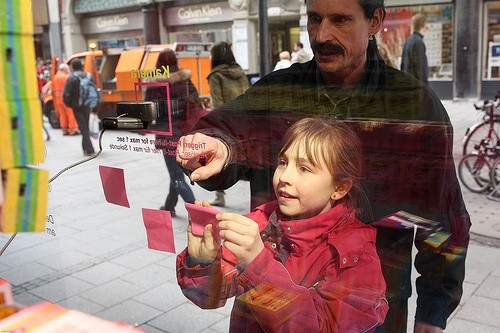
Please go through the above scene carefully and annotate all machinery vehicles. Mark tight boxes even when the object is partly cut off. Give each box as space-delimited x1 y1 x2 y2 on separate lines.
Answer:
40 43 212 129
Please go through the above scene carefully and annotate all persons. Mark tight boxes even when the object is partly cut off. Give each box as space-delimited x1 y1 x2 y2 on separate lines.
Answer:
175 0 472 333
400 11 431 85
176 116 389 333
377 43 397 70
143 41 312 217
33 56 54 103
62 59 100 155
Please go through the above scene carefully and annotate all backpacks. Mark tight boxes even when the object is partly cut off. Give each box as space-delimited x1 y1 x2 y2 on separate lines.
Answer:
73 72 98 112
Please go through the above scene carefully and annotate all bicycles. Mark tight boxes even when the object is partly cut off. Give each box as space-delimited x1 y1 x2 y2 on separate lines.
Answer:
457 97 500 197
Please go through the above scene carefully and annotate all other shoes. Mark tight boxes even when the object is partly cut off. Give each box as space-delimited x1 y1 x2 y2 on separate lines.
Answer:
82 147 94 154
46 135 51 142
160 206 176 218
62 129 81 136
207 198 225 207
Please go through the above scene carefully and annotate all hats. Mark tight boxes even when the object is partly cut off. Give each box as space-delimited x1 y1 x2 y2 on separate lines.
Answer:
280 51 290 59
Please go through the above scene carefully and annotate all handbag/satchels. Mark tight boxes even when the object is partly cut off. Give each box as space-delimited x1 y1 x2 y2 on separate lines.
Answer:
185 79 208 128
